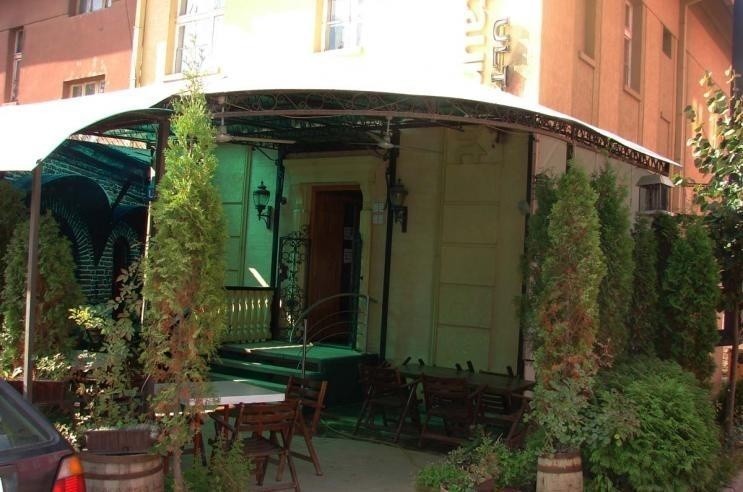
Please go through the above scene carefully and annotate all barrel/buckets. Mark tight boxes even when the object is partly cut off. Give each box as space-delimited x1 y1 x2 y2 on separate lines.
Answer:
80 452 168 492
535 449 584 491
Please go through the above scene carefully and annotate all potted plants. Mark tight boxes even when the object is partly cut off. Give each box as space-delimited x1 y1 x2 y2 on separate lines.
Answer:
524 373 587 492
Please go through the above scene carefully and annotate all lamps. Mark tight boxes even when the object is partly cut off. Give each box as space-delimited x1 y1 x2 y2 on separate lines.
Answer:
251 183 272 230
388 179 408 232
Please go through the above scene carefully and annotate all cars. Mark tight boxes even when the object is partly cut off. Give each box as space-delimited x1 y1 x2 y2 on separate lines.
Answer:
0 376 86 492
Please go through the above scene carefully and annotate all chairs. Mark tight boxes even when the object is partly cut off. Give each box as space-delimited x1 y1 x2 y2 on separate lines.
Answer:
148 375 328 491
351 355 535 457
